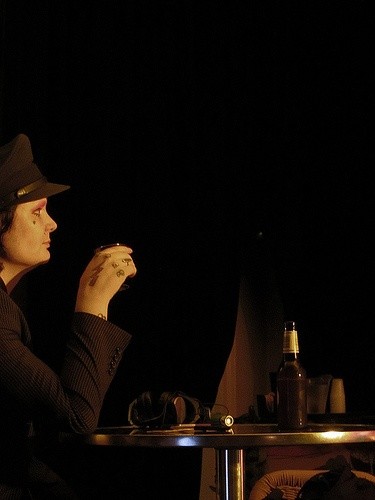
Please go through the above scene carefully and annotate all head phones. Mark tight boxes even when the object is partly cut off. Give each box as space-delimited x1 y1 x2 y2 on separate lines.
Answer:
128 390 186 428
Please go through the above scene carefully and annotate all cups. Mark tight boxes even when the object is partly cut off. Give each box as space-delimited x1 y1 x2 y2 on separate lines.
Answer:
308 377 346 424
94 242 129 291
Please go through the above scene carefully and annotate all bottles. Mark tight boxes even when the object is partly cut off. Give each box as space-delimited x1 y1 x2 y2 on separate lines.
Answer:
275 320 307 430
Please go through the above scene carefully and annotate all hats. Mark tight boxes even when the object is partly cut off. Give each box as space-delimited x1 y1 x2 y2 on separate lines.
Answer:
0 133 71 207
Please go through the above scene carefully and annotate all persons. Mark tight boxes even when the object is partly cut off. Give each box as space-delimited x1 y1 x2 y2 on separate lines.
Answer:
0 132 138 500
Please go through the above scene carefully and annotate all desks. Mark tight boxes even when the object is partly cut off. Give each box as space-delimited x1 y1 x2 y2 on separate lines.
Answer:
93 423 375 500
